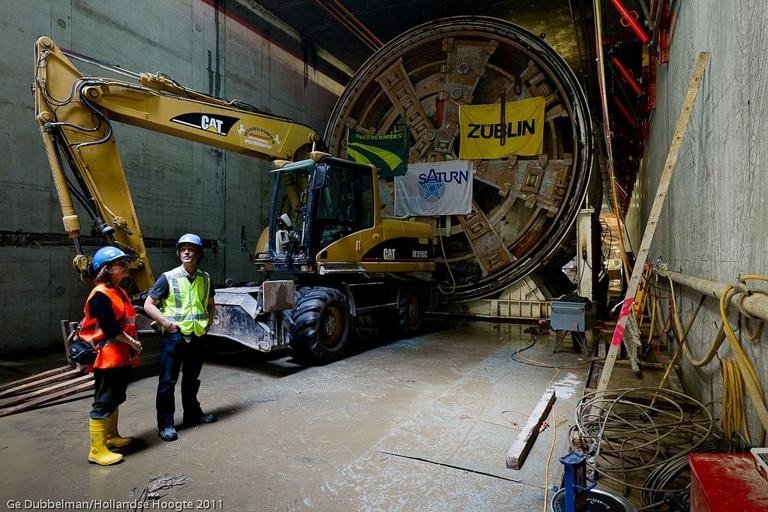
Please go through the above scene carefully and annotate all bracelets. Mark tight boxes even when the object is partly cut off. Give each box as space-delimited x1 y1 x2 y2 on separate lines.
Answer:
127 336 134 346
166 322 172 329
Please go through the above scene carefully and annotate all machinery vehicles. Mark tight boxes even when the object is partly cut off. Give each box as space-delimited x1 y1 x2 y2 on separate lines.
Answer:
27 33 438 367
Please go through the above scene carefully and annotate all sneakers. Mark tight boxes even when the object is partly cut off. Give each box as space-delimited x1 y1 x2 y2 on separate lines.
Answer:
183 414 215 426
160 424 177 441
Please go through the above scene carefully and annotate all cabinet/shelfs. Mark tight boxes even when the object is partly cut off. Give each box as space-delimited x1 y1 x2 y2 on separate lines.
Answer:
688 453 768 512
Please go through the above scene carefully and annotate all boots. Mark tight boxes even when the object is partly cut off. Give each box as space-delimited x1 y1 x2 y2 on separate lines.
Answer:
88 408 133 466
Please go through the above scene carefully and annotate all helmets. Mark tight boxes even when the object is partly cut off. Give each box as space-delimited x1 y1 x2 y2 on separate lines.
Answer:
92 246 129 270
175 234 203 254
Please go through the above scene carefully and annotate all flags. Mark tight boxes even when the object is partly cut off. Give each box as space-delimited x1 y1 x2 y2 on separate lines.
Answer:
344 129 409 180
390 158 476 219
454 94 546 161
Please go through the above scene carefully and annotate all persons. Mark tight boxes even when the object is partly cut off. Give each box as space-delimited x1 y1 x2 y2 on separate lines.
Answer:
142 231 218 442
77 244 145 469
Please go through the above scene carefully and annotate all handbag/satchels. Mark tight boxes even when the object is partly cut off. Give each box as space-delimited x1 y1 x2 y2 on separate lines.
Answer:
69 342 96 365
